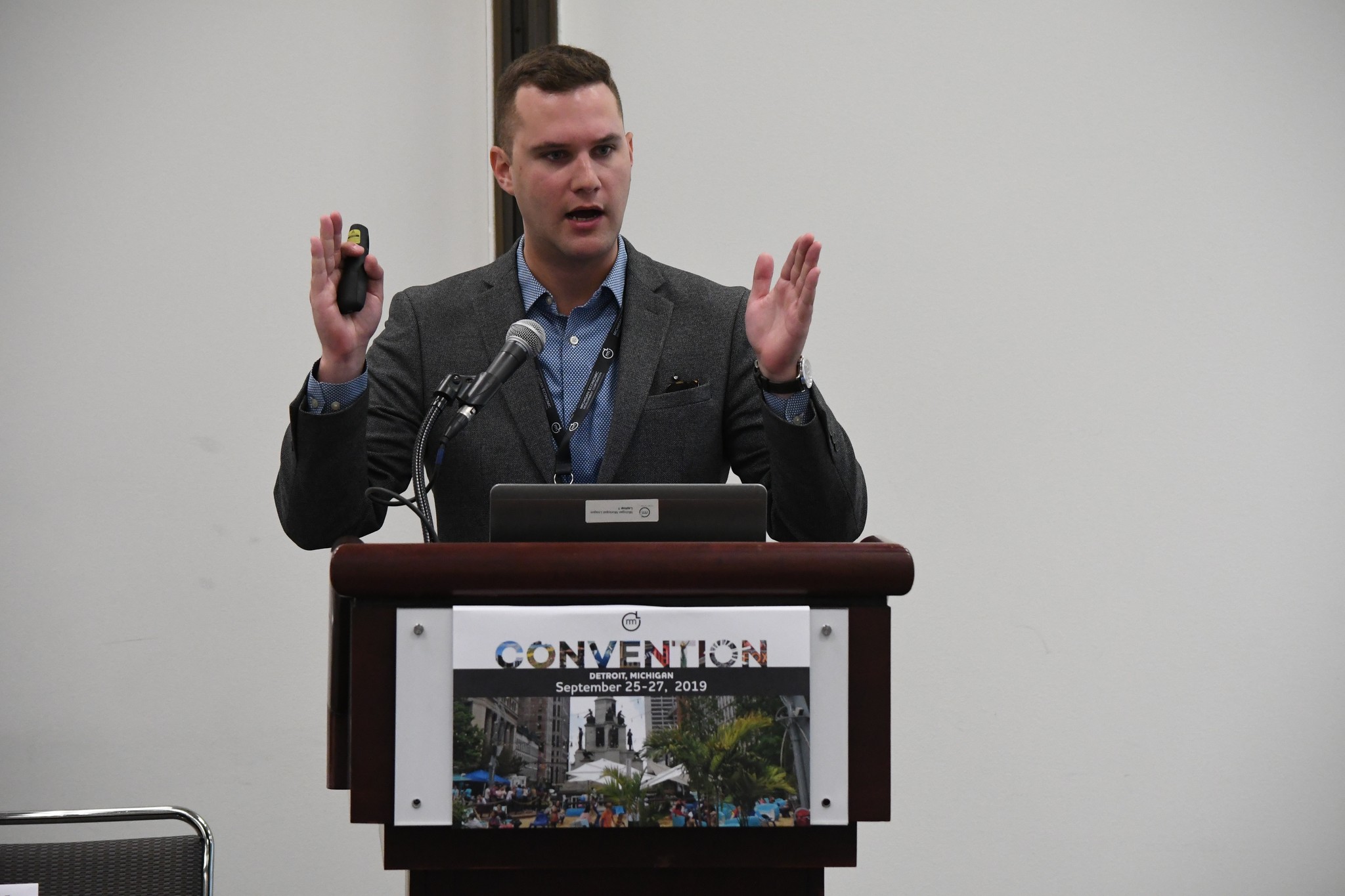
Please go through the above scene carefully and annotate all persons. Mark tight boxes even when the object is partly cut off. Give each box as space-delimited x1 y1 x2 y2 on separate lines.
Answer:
271 47 872 553
575 705 633 761
452 784 812 826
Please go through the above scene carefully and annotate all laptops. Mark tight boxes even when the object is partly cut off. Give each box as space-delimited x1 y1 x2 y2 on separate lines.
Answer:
488 483 768 542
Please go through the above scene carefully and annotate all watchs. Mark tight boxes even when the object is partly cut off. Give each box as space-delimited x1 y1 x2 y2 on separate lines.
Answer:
750 355 814 394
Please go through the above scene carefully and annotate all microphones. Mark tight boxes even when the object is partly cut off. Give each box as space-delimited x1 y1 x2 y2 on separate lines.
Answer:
439 319 546 446
